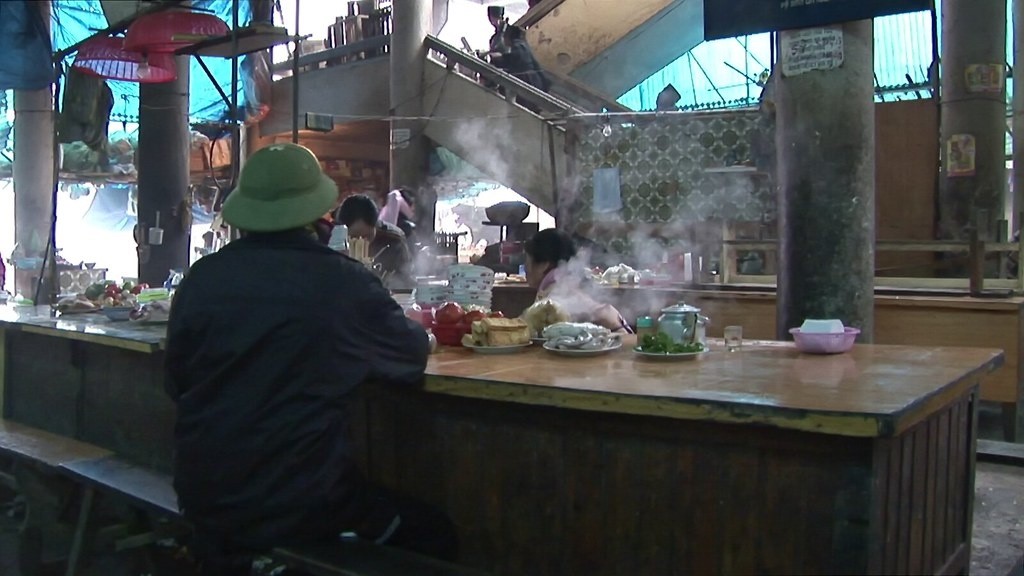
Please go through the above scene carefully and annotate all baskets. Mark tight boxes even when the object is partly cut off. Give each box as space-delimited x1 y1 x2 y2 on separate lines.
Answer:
789 327 861 354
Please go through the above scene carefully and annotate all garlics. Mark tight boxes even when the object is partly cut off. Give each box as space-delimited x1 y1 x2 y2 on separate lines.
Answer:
105 289 136 307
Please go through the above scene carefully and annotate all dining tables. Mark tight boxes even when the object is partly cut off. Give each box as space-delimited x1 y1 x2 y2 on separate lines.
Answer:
1 298 1005 576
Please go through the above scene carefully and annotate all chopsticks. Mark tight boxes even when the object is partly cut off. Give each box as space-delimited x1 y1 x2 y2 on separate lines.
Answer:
349 237 369 260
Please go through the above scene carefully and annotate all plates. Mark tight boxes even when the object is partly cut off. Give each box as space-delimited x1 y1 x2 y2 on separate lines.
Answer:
633 346 709 358
462 337 622 356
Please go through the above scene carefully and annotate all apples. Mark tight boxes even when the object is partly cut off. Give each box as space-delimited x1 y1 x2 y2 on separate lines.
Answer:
107 282 149 297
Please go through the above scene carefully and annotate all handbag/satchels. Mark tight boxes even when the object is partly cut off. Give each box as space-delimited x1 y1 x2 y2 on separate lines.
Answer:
530 52 552 92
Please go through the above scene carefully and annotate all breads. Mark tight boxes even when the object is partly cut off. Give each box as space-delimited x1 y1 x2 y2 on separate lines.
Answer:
460 319 529 346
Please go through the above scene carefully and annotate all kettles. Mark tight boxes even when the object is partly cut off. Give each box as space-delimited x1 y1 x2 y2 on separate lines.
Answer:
656 299 709 344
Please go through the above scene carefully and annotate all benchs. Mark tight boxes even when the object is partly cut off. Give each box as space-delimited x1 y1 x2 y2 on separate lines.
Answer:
1 416 493 576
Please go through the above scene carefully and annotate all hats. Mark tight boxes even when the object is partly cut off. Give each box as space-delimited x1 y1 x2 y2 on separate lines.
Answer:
221 143 339 231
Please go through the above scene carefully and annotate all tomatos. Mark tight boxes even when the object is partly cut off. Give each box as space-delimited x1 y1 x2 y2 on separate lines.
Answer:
436 303 503 322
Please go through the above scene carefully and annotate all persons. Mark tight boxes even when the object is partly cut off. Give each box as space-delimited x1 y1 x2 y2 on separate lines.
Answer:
162 143 459 564
475 6 551 115
378 184 418 260
337 194 418 289
522 228 634 334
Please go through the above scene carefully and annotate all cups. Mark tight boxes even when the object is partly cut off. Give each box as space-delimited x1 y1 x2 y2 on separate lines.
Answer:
149 228 163 244
636 316 654 345
723 325 743 349
332 224 348 250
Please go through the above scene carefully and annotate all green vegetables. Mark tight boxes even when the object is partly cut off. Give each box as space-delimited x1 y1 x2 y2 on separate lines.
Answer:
641 333 705 354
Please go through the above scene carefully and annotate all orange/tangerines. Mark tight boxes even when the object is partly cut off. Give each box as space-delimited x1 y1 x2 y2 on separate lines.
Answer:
85 283 103 299
123 281 135 292
104 280 112 289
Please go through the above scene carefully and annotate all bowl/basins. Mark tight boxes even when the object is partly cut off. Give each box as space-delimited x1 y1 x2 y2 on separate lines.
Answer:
789 327 860 353
415 263 494 309
85 262 95 269
485 205 530 223
432 324 471 347
103 306 135 321
